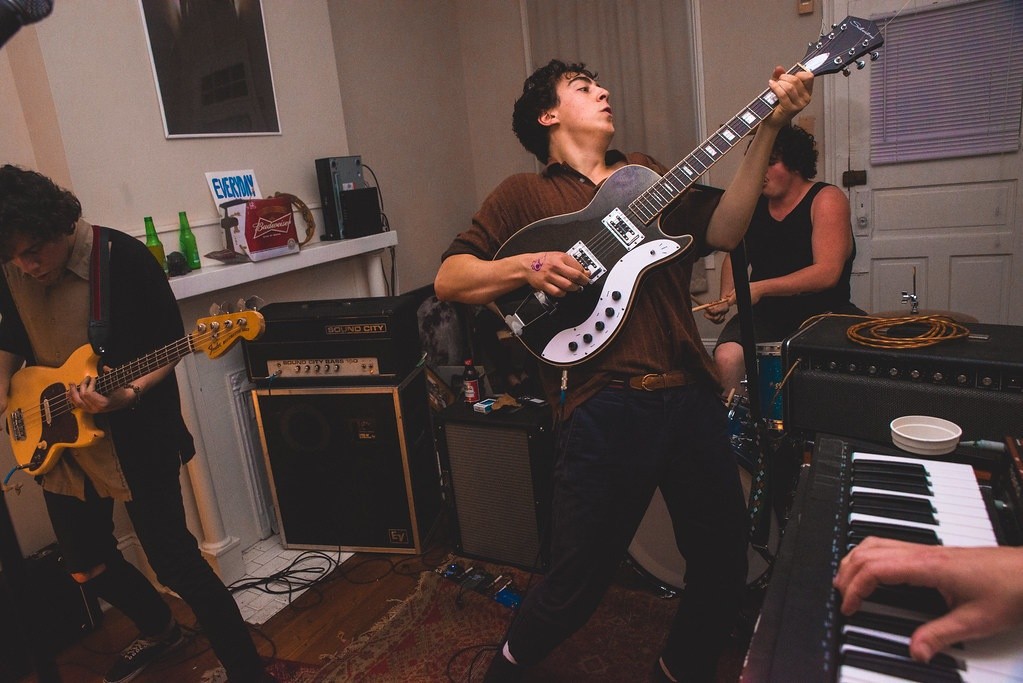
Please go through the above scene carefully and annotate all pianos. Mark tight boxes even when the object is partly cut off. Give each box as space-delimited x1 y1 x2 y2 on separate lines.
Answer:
737 429 1023 683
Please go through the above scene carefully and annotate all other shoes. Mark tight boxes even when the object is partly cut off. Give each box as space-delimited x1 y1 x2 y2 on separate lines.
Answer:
657 643 713 683
483 648 520 683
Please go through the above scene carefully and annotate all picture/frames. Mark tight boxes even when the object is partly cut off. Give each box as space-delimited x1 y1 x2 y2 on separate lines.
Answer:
137 0 282 140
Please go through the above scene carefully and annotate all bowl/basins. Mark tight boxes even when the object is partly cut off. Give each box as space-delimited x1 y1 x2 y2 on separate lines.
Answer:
890 416 962 456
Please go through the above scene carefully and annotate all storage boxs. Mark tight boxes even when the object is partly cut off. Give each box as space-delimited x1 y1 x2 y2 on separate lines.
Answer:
473 398 496 414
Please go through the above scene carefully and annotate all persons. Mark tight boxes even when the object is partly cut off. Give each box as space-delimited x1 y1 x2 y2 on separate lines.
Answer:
0 164 265 682
836 536 1023 664
434 59 814 683
703 123 857 398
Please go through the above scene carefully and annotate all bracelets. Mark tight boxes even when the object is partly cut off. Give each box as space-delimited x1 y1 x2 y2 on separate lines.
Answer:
124 383 141 412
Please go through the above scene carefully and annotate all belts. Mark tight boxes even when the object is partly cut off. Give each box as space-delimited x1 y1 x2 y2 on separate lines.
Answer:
604 368 701 392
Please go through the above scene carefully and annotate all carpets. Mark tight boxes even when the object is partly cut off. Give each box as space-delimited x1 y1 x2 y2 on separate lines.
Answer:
202 652 318 683
302 549 744 683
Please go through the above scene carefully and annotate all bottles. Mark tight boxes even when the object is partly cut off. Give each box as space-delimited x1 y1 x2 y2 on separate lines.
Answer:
144 217 170 280
462 359 480 407
178 211 201 270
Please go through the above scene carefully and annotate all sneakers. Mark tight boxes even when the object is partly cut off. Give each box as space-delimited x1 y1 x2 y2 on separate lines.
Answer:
103 617 185 683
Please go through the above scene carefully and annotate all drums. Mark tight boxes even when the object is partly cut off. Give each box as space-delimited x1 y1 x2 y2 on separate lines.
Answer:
755 341 784 432
622 387 780 601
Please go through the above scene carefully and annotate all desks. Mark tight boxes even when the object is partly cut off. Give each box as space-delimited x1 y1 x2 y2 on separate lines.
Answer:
167 229 399 588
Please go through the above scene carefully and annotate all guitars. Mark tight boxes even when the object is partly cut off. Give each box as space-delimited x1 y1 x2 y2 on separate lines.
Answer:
491 14 887 368
5 294 266 477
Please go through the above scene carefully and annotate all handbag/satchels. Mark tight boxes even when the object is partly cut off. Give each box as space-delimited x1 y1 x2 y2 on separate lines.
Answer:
228 194 300 262
